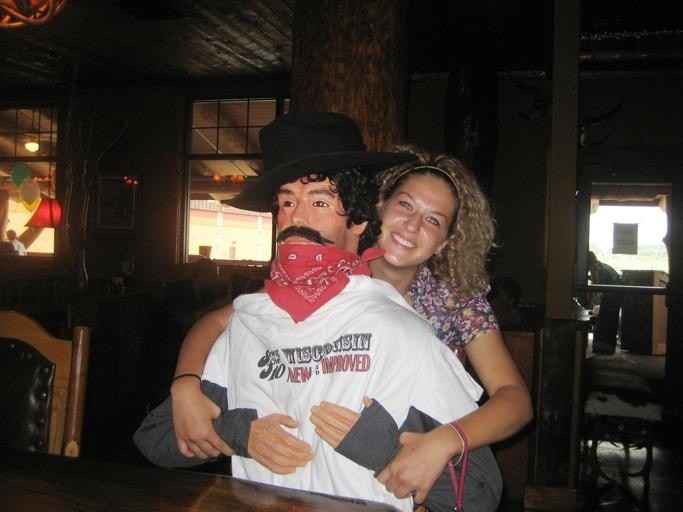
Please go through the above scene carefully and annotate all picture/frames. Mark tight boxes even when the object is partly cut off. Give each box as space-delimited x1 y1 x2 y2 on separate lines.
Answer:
90 165 142 241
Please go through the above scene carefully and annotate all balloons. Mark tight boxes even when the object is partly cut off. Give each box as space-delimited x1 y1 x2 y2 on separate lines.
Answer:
11 164 40 212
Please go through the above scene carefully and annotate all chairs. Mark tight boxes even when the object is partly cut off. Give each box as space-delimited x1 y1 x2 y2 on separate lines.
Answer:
0 308 92 457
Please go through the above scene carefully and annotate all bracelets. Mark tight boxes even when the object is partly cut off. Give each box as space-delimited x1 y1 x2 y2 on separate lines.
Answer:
447 421 466 467
171 373 202 383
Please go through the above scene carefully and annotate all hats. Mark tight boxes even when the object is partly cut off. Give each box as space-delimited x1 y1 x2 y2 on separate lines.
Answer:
219 109 420 213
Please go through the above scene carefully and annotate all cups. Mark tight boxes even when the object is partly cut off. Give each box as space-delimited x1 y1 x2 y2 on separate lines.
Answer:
198 246 213 258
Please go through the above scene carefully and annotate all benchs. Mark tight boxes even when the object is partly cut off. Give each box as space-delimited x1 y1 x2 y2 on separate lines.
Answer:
582 354 664 483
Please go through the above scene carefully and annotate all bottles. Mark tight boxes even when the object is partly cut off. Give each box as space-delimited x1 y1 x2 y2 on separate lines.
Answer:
228 241 237 259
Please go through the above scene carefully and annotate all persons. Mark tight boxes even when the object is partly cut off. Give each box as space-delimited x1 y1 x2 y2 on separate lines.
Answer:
7 229 28 256
132 108 504 512
587 250 623 355
168 141 534 505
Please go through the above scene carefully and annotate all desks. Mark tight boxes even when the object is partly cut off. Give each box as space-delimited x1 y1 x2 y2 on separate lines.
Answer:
0 448 397 511
